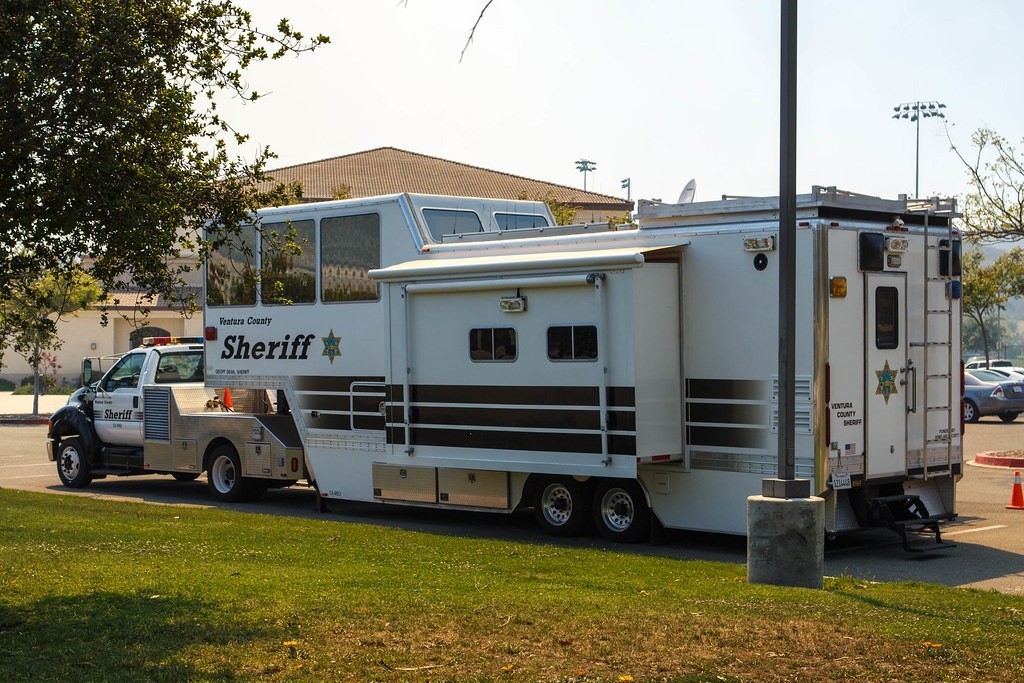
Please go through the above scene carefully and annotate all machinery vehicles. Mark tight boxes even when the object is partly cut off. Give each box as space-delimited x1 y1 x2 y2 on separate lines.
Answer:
46 335 303 500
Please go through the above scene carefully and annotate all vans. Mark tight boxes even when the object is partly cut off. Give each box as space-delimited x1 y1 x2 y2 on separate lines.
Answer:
962 359 1014 368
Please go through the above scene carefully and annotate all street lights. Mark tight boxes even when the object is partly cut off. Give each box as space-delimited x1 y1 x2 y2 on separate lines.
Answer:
892 99 946 199
575 157 597 193
620 175 632 202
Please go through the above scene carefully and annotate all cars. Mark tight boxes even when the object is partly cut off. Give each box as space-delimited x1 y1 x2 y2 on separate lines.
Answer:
964 367 1024 422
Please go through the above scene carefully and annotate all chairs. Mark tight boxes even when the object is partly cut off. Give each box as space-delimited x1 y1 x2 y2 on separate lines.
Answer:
160 364 181 380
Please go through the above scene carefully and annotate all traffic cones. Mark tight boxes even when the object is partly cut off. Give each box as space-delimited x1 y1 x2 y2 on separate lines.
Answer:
222 388 234 412
1005 468 1024 509
